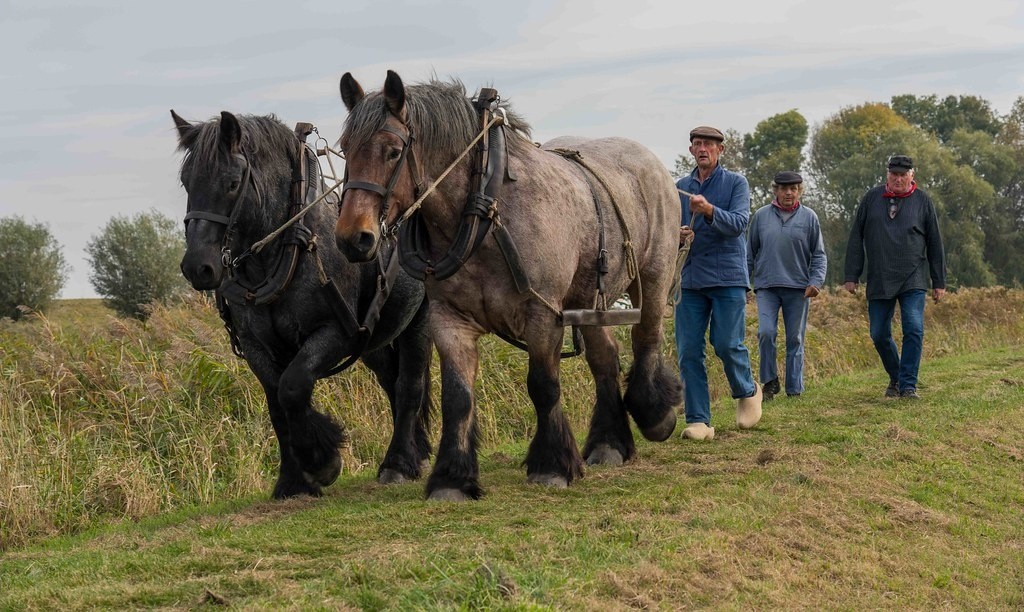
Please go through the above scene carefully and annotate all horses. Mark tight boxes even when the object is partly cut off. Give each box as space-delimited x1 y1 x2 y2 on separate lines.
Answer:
169 108 434 501
333 69 684 510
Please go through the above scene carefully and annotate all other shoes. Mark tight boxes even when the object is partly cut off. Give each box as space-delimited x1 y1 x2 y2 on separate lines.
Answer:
762 377 782 401
786 393 799 398
902 387 922 401
680 422 715 441
736 379 763 430
885 380 900 399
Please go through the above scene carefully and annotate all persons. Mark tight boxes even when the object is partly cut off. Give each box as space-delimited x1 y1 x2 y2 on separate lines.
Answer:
747 172 826 403
845 155 947 398
676 127 762 443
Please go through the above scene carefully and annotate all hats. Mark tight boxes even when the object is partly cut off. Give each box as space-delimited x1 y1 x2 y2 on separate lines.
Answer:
773 171 803 185
887 154 914 173
689 126 725 142
